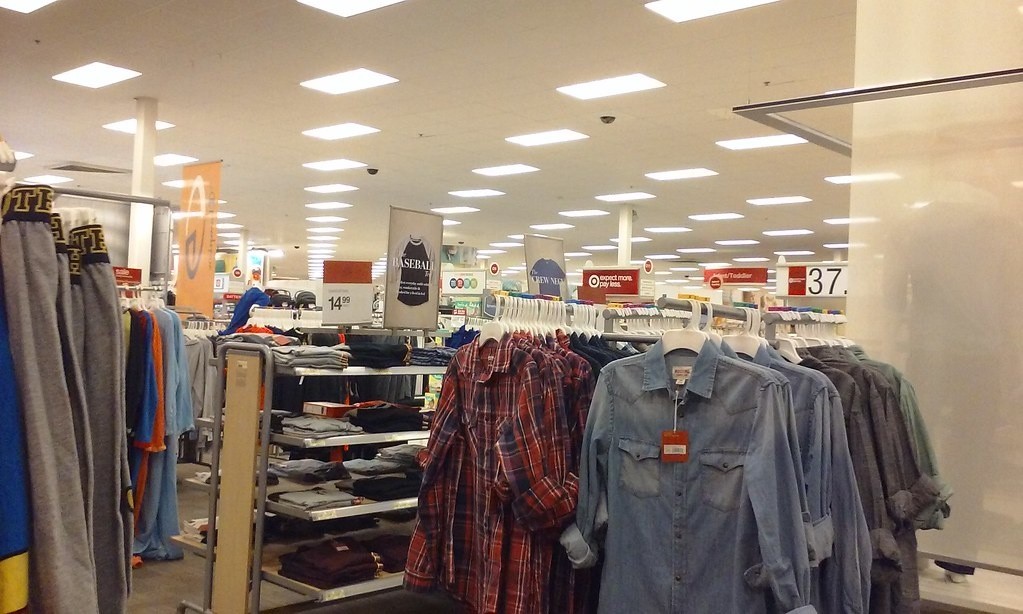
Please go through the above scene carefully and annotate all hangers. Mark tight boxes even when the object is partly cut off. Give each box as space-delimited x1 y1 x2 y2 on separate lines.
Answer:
117 281 869 371
0 138 97 228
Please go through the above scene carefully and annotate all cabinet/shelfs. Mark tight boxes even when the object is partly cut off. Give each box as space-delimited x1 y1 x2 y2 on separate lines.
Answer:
171 335 448 614
